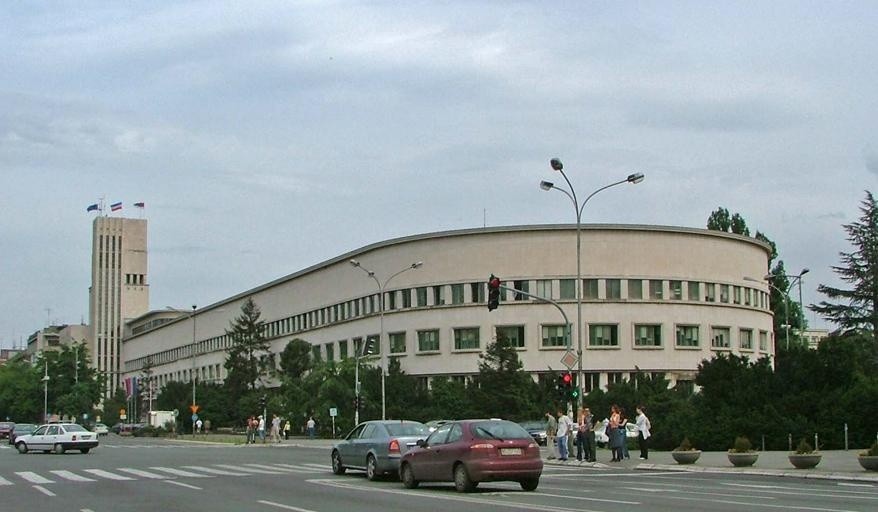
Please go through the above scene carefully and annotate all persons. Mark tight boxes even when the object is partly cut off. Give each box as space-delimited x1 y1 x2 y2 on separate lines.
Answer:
196 414 316 443
544 403 651 462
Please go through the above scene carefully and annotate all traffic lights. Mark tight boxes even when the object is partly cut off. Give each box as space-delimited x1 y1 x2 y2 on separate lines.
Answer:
258 397 266 409
487 274 500 313
364 337 376 356
559 373 570 387
568 388 579 400
357 395 367 411
352 394 357 411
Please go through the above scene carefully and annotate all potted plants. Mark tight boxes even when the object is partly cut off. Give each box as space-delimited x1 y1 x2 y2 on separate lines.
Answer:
669 435 877 471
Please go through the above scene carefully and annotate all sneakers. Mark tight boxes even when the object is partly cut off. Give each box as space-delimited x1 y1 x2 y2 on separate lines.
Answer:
547 455 596 462
639 457 646 459
624 456 629 459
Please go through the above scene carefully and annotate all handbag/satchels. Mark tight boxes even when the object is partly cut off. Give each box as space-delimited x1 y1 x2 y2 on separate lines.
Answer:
645 417 652 429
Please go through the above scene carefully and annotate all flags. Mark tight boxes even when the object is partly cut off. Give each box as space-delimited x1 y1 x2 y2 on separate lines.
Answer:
124 377 136 401
87 203 98 211
110 202 123 211
133 201 145 208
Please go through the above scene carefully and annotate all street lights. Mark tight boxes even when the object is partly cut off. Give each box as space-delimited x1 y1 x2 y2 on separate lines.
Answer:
347 256 427 421
762 274 804 349
537 156 646 417
166 304 197 438
40 376 50 424
743 267 810 352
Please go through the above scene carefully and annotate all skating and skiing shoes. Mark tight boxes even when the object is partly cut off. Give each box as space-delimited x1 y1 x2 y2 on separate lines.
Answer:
609 457 620 463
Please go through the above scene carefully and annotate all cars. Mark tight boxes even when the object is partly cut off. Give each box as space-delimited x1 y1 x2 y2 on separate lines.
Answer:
417 418 642 446
13 423 100 456
396 417 544 495
324 419 434 482
0 420 144 444
